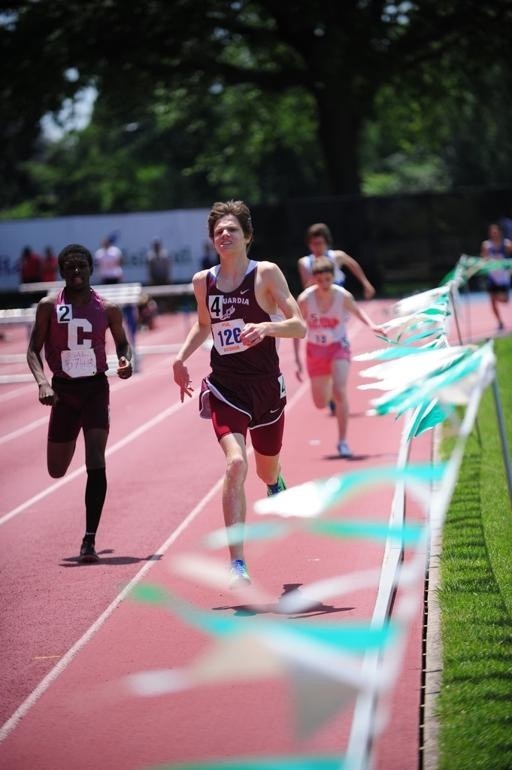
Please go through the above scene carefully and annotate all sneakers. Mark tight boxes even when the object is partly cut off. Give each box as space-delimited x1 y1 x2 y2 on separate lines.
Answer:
79 540 100 562
227 560 251 589
268 473 286 499
337 442 350 457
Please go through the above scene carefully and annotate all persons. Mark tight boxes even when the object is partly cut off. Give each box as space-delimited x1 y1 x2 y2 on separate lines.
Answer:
38 245 59 281
482 222 512 332
293 257 389 458
26 243 133 563
96 227 123 285
145 238 172 285
201 242 220 274
173 200 308 587
18 240 43 308
134 292 157 332
298 223 374 301
